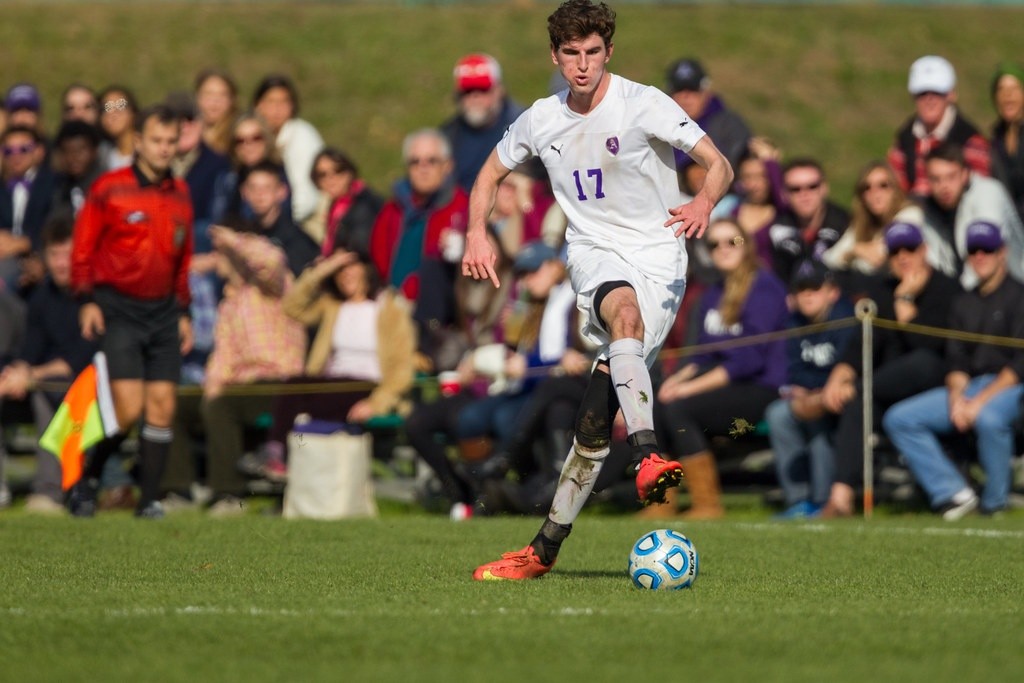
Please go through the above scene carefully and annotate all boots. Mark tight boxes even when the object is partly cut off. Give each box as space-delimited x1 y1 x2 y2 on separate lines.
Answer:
635 452 725 520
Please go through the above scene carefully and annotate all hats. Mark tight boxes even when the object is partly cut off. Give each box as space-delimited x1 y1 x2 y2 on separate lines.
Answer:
7 83 40 112
907 56 957 93
966 222 1000 248
454 52 503 91
792 258 829 293
665 57 708 95
887 223 924 249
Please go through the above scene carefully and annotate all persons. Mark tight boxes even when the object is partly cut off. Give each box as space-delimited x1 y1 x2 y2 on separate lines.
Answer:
462 0 734 581
0 54 1023 523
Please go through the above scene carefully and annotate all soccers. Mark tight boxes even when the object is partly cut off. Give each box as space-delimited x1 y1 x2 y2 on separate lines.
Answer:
628 529 700 590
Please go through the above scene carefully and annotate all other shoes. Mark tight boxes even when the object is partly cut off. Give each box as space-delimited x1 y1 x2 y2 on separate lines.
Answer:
71 484 98 515
782 500 822 519
817 502 854 521
941 489 978 521
134 500 165 522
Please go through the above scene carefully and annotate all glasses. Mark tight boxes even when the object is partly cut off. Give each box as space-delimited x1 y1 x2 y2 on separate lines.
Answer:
3 143 36 158
889 244 917 256
859 181 893 193
318 167 345 177
234 133 264 146
407 157 444 168
967 246 997 255
707 236 745 251
788 176 823 192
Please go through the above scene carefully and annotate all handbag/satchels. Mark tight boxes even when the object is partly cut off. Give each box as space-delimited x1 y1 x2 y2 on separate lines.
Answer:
280 413 384 521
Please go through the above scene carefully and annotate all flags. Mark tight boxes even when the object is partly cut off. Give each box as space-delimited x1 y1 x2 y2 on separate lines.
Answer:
40 351 117 492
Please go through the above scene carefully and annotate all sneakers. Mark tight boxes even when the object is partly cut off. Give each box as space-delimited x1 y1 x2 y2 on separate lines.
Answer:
473 544 557 582
636 452 684 504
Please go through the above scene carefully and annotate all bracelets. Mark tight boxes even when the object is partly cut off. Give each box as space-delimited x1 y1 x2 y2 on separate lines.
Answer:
894 292 914 301
